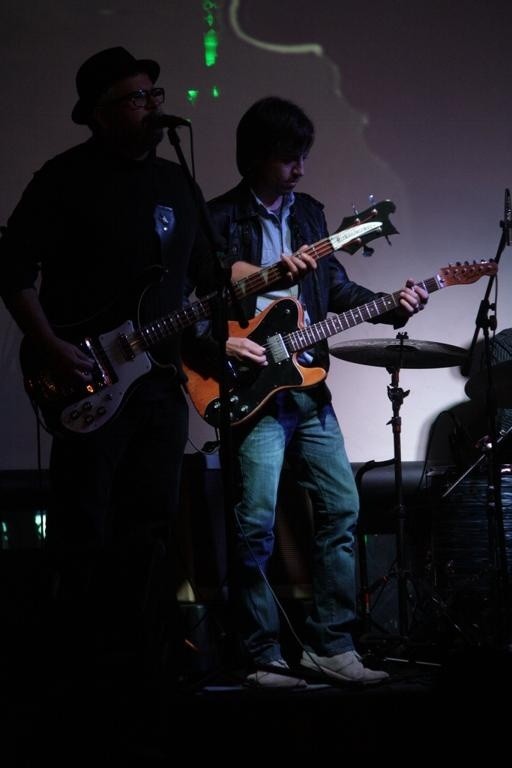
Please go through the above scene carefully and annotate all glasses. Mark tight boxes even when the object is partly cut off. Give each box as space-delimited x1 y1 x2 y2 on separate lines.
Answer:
92 88 165 110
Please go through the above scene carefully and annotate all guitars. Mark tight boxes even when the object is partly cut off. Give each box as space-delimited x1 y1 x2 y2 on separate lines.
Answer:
19 195 398 431
174 254 500 430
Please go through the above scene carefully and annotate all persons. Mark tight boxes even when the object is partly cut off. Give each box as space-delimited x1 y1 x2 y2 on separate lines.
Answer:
204 97 430 689
1 48 318 683
425 338 512 471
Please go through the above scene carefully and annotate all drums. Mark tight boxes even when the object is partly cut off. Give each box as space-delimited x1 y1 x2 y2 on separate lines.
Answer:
426 462 512 606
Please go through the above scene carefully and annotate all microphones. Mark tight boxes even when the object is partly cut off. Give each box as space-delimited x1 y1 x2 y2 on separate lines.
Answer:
145 112 191 129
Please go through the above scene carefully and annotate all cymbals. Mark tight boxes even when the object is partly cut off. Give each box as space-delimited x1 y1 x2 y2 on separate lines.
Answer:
326 338 468 370
466 358 511 411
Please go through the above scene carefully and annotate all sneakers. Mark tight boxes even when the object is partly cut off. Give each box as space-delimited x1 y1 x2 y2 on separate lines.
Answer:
246 644 390 688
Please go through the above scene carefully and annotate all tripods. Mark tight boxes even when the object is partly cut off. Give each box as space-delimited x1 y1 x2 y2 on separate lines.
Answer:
151 131 512 685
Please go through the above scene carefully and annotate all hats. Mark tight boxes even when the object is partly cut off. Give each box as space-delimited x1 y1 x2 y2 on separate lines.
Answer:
72 46 160 125
